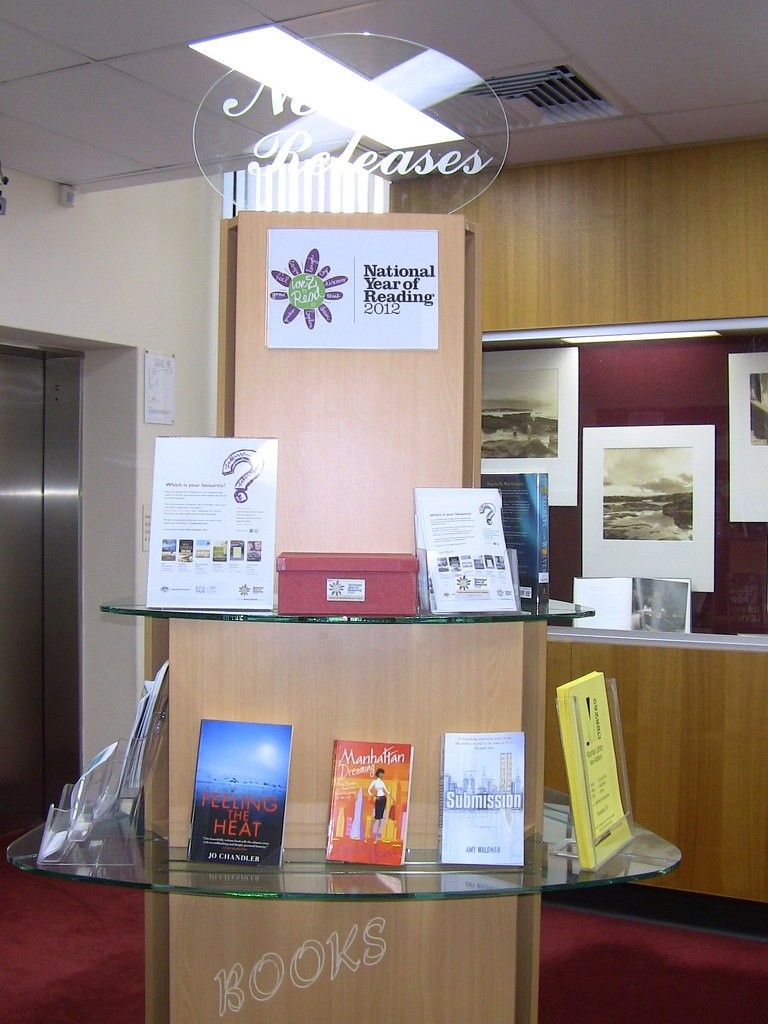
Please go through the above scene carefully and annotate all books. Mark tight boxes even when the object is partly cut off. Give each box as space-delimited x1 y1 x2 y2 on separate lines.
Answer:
481 473 549 616
325 739 414 866
128 661 168 787
438 732 525 866
188 719 293 868
573 576 692 634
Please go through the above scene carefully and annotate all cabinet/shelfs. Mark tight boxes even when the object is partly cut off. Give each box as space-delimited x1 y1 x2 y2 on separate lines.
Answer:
6 600 684 902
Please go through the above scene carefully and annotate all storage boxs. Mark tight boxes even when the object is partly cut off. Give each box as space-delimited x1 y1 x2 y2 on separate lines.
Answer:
275 551 419 616
